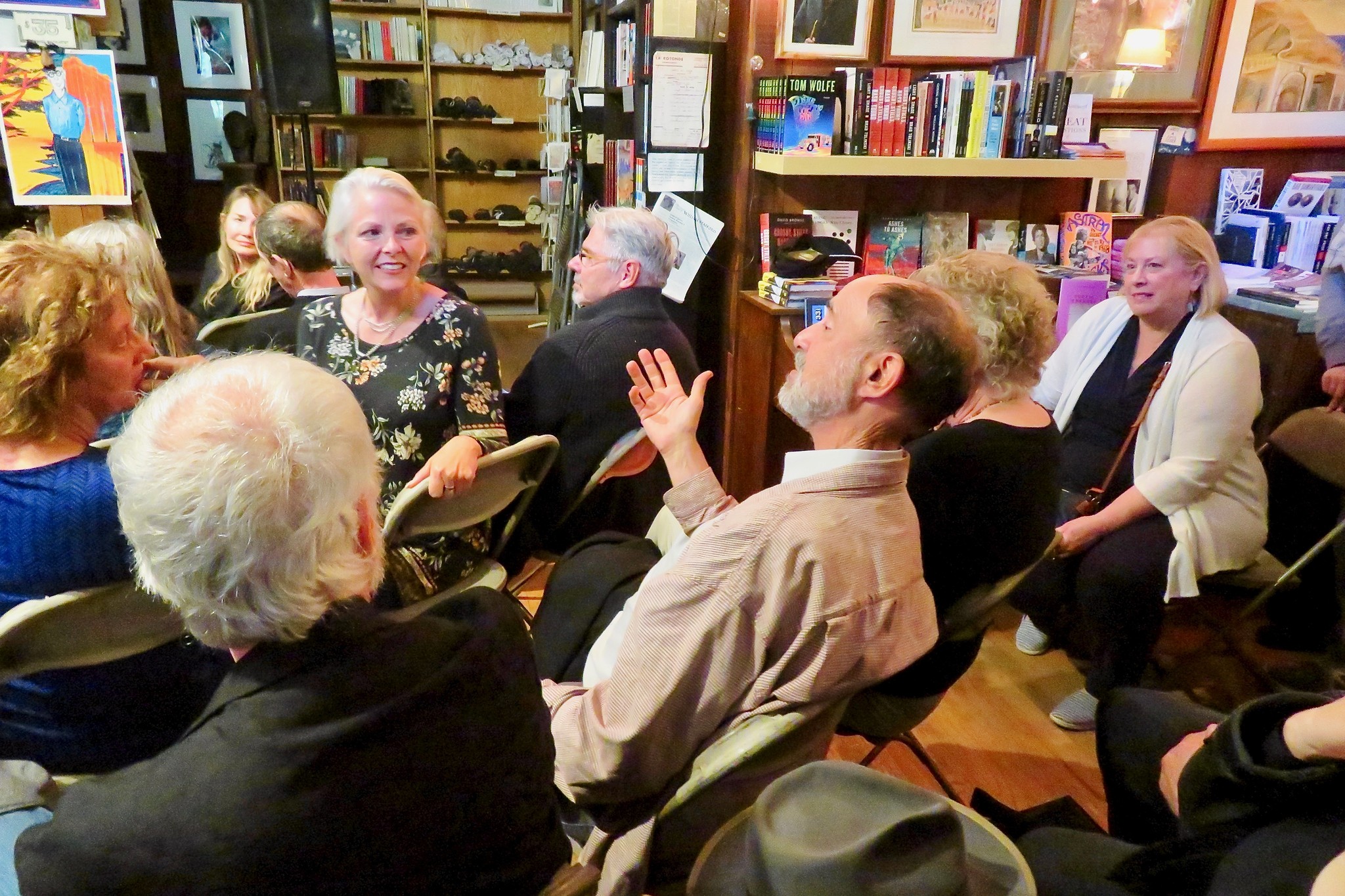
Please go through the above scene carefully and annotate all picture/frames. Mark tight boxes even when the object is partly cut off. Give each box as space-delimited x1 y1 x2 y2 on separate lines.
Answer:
774 0 874 59
1198 0 1345 151
1034 0 1224 115
883 0 1029 64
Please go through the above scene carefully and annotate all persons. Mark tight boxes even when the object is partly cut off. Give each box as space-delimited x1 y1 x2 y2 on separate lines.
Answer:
192 19 234 73
0 168 1345 896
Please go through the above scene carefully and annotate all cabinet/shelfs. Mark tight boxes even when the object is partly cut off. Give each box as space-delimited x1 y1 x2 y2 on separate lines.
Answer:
567 0 644 214
424 0 579 313
1222 293 1324 446
751 156 1125 327
267 0 433 321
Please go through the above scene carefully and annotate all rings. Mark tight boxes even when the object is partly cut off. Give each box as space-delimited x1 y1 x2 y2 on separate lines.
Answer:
445 486 455 490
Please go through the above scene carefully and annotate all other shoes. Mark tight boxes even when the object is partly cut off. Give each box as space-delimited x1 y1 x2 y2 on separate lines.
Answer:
1050 687 1100 731
1016 610 1051 656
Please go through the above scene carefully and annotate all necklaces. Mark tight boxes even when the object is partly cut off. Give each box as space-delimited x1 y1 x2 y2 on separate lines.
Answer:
354 289 418 357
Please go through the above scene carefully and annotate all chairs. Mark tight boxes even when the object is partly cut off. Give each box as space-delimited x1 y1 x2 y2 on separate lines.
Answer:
0 436 1345 896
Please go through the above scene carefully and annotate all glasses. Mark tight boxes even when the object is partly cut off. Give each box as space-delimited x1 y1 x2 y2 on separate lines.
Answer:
577 248 629 265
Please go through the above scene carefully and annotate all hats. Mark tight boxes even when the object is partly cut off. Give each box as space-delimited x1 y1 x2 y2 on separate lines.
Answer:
771 235 862 278
684 758 1036 895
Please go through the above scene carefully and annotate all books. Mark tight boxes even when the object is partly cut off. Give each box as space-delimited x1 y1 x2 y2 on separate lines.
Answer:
277 124 357 219
748 55 1345 324
534 18 646 269
334 16 418 116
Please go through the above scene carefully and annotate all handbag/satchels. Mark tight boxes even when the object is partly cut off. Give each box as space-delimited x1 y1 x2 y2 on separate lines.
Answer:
1053 488 1103 516
